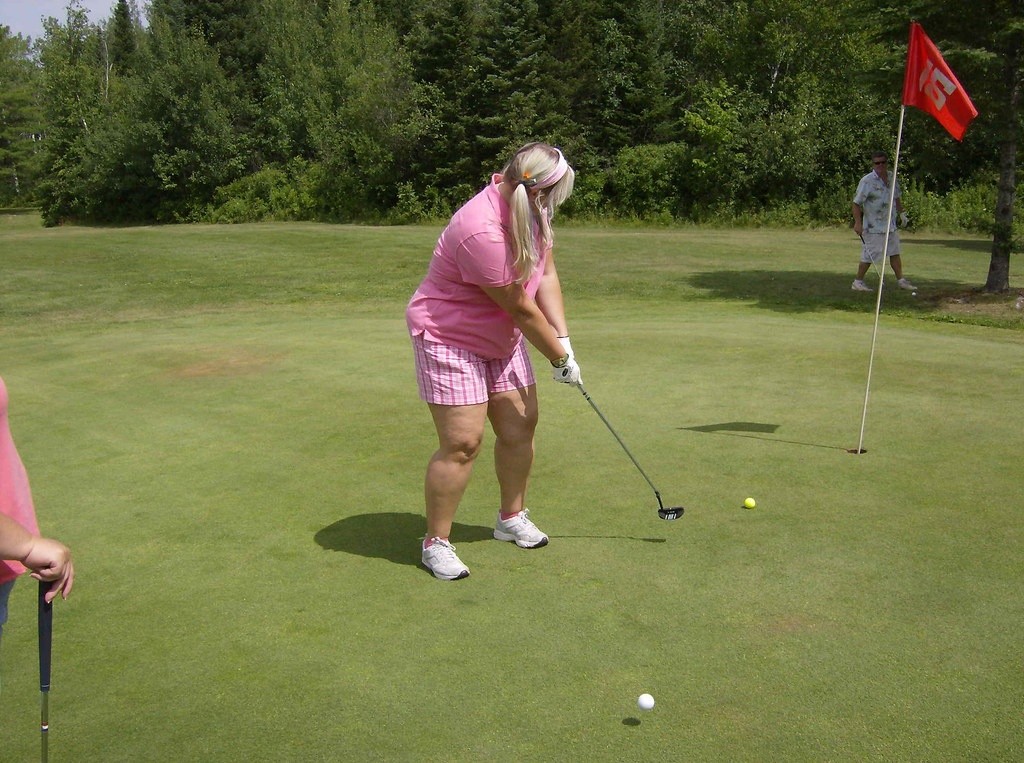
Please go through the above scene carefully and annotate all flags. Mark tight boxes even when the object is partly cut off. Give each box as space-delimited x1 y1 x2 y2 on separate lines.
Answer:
900 18 978 141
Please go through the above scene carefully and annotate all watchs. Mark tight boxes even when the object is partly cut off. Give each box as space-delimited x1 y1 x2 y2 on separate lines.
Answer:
552 355 568 367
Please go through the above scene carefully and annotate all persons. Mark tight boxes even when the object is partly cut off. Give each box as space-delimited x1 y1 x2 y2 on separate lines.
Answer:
0 376 75 626
851 151 918 292
405 142 584 580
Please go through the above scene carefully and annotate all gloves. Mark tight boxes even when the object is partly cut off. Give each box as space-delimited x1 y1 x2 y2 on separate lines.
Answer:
900 214 909 227
552 337 583 387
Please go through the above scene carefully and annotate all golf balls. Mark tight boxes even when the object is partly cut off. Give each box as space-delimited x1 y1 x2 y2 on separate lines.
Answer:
744 497 756 509
638 693 655 711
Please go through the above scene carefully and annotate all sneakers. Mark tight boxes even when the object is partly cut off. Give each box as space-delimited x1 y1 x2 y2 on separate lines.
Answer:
422 533 470 580
899 280 917 290
494 508 549 548
852 281 872 292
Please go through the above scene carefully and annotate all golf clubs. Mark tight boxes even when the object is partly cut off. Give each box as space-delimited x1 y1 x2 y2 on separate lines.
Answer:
860 234 885 285
37 581 53 763
575 380 685 520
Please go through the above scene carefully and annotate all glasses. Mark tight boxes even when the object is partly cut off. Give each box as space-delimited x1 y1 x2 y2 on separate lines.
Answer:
874 161 886 165
